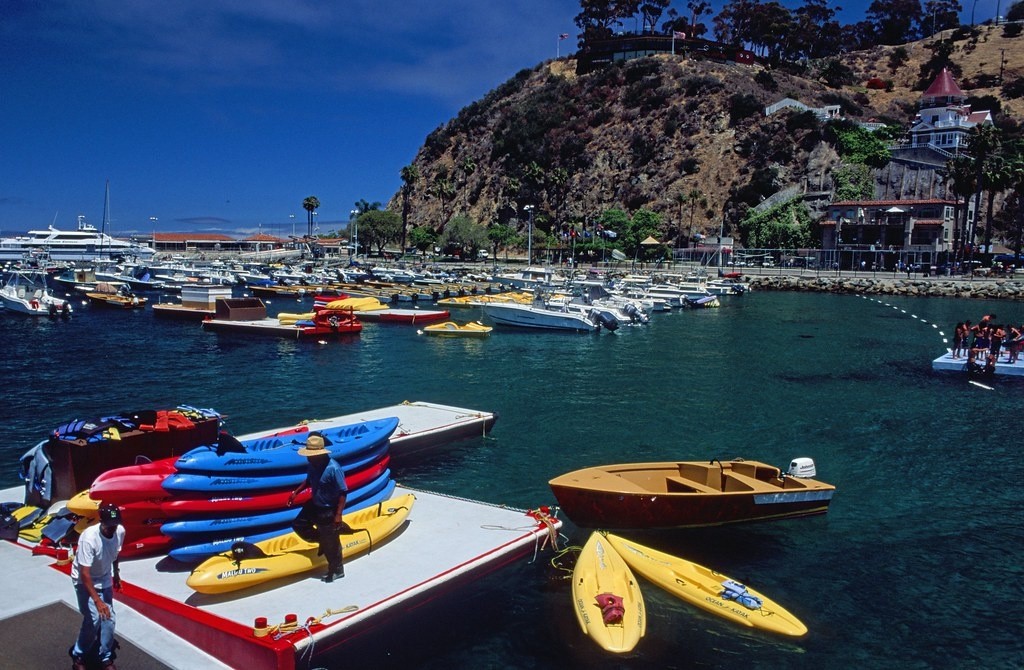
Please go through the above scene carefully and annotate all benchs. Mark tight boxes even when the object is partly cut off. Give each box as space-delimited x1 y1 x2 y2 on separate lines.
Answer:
665 476 722 493
725 471 785 491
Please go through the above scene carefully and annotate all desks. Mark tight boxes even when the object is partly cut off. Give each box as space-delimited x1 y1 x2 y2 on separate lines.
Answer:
49 413 227 505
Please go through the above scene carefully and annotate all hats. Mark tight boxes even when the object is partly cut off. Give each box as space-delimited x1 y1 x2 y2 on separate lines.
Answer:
99 505 122 526
298 436 330 456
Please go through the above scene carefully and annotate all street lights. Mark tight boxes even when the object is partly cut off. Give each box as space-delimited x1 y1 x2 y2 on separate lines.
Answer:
289 215 295 237
351 210 358 258
523 205 535 266
312 212 318 235
148 217 159 249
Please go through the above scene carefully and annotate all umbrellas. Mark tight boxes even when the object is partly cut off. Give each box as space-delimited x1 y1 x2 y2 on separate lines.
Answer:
886 207 904 217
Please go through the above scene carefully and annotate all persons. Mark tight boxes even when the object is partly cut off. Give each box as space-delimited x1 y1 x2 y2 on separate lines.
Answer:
605 258 609 266
68 504 126 670
566 256 572 266
952 313 1024 379
900 260 904 273
950 265 956 278
288 436 348 582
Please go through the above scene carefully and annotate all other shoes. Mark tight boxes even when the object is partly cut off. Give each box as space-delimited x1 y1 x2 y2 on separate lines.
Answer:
98 659 116 670
69 644 87 670
317 542 325 557
322 569 345 583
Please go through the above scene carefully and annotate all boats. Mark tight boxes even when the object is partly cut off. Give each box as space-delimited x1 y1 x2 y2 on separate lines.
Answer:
571 530 648 654
0 179 753 337
548 457 836 530
605 533 809 637
65 417 416 595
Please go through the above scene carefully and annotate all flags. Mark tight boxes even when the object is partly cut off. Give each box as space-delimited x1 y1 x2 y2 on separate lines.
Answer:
559 34 569 40
617 21 623 26
673 31 685 39
562 230 590 238
